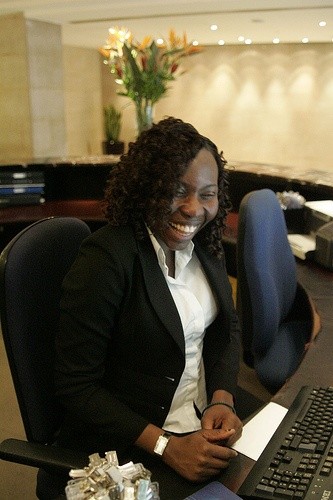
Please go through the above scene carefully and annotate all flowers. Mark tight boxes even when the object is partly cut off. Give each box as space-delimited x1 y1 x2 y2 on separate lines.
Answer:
98 25 204 106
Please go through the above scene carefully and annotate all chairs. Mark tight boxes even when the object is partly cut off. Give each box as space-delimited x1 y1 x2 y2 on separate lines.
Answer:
235 186 312 394
0 216 160 500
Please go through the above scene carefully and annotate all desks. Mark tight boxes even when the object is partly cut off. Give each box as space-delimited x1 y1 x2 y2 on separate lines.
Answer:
0 200 333 500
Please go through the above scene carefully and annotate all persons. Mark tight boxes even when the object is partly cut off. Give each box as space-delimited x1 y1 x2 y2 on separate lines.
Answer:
54 117 243 481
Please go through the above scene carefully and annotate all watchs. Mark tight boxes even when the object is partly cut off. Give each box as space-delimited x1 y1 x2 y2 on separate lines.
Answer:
152 431 172 462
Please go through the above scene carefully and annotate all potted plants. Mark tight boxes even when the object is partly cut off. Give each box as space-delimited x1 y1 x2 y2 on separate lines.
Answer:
102 107 126 155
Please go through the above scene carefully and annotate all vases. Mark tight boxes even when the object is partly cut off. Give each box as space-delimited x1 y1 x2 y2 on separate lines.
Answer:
135 107 153 139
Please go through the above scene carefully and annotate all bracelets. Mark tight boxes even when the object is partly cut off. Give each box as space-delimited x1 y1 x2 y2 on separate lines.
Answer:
202 402 236 416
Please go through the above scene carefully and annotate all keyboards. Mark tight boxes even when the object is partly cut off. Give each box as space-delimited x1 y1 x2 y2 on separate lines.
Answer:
237 384 333 500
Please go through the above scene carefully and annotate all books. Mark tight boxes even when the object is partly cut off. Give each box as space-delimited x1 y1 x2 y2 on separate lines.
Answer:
0 171 46 205
